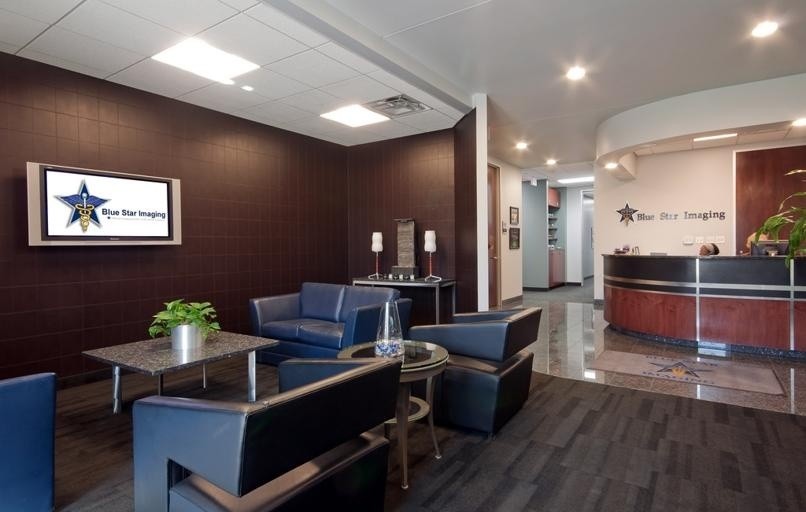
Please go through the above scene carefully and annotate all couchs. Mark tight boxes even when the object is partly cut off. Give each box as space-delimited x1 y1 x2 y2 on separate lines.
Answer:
249 282 413 366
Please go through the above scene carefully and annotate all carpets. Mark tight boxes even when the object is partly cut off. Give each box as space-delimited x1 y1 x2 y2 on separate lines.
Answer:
587 349 788 397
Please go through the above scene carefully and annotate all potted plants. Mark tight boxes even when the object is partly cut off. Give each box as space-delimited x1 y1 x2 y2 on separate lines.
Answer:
148 299 221 352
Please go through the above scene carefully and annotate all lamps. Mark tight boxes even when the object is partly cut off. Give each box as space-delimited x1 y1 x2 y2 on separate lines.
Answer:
424 230 442 282
368 231 383 280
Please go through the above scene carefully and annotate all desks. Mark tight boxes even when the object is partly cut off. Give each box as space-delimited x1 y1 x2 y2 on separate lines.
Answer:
352 277 456 325
337 340 449 489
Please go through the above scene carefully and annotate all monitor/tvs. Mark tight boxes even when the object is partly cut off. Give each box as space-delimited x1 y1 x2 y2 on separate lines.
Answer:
750 240 790 255
26 161 182 247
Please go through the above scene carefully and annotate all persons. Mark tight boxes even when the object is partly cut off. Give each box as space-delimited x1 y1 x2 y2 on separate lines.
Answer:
742 232 767 255
699 243 720 255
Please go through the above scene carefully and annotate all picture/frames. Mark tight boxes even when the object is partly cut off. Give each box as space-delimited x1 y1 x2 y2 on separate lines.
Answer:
510 206 519 225
508 227 520 249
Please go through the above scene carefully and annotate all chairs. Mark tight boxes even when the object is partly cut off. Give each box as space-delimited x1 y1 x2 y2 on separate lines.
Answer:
407 306 542 440
131 358 403 512
0 371 56 512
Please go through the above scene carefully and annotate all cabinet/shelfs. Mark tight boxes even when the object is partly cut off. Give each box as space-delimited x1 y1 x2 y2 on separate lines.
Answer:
548 187 565 287
